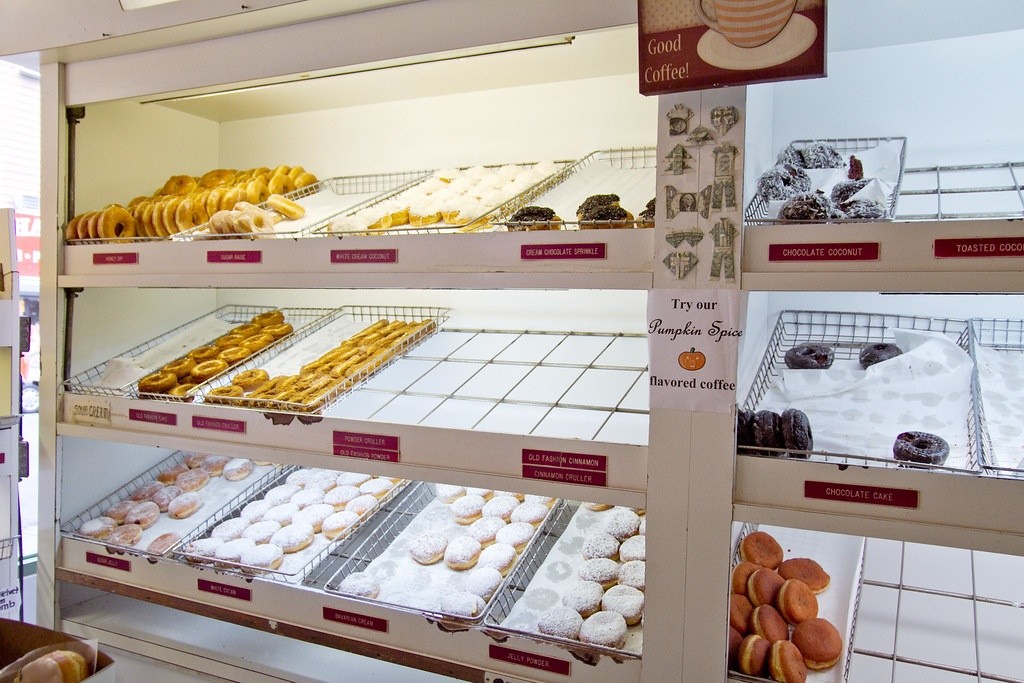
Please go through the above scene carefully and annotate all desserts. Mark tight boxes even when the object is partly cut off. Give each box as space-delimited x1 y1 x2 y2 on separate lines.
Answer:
325 137 894 241
75 450 843 683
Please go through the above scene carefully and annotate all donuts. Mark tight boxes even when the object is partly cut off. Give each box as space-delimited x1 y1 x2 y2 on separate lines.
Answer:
738 339 951 469
63 163 437 413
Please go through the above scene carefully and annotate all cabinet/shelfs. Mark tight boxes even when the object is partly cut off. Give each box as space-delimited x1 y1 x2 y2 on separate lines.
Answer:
0 0 1022 683
